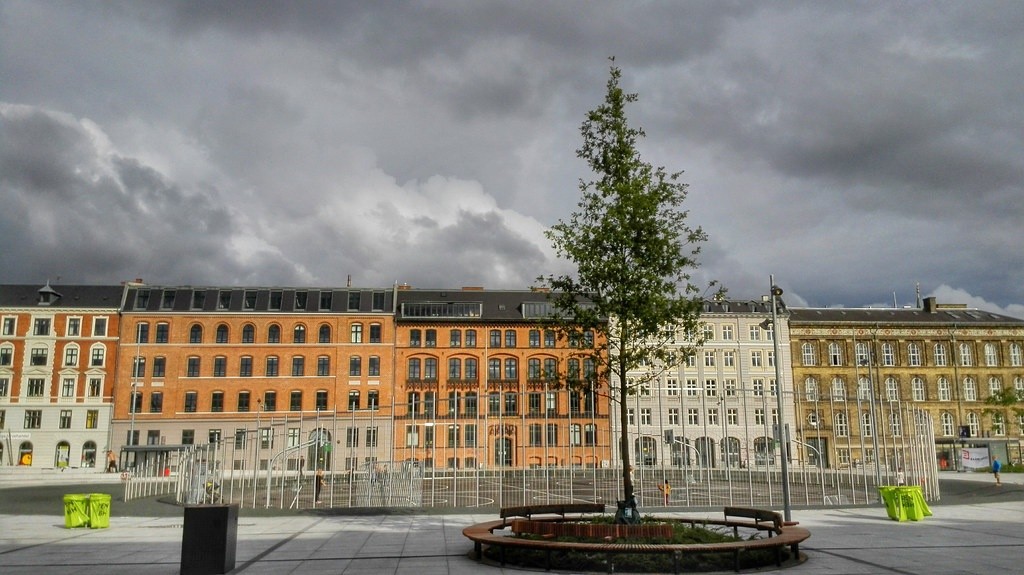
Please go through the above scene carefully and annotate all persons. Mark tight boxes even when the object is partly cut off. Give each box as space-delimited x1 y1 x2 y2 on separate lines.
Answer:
629 465 635 494
992 455 1002 486
314 467 326 504
897 467 905 486
106 450 119 473
203 477 224 505
299 455 306 476
658 479 670 506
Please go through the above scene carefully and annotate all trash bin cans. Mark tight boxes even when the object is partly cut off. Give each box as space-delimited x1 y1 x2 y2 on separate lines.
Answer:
63 493 111 530
179 503 239 575
878 485 932 522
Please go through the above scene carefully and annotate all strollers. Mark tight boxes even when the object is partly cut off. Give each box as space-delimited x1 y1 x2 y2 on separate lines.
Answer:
197 481 227 505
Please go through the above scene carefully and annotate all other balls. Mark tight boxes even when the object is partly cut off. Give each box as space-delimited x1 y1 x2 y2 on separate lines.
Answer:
337 439 340 444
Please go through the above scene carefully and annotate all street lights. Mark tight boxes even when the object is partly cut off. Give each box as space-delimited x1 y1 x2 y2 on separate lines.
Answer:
760 273 800 524
790 436 828 506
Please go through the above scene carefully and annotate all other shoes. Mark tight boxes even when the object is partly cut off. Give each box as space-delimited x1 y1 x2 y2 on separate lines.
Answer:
996 484 999 485
998 484 1001 486
317 500 322 504
662 503 665 505
667 503 669 504
312 501 317 504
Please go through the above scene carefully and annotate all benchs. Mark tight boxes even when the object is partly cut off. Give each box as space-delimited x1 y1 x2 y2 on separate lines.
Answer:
493 503 605 530
723 505 801 535
